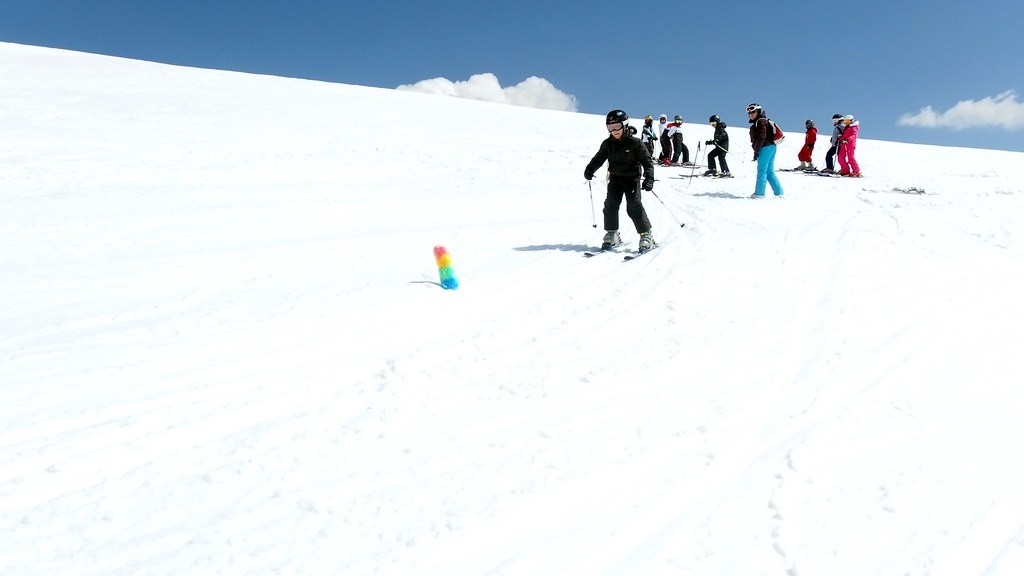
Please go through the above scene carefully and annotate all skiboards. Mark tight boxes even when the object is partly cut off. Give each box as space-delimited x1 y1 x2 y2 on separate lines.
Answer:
778 168 863 178
659 161 700 169
585 238 666 261
669 173 735 179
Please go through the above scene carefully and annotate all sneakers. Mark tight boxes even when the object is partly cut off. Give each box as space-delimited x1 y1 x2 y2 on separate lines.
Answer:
603 229 617 246
639 229 651 248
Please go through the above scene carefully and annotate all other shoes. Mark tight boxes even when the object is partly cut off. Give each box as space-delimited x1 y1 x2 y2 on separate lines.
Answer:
703 168 730 176
650 155 694 168
793 162 859 178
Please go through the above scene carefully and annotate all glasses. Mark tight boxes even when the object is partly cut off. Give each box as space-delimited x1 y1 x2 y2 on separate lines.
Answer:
607 122 622 131
710 121 717 126
746 106 755 113
805 124 811 128
844 120 852 125
832 118 839 123
676 119 682 124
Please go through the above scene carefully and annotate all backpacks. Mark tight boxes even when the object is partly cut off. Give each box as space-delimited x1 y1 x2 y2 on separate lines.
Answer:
756 117 785 142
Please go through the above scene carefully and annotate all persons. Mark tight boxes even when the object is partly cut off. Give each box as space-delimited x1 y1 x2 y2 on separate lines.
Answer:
794 120 818 171
638 115 658 164
656 114 695 166
819 113 861 178
745 103 787 198
704 114 731 177
583 109 655 250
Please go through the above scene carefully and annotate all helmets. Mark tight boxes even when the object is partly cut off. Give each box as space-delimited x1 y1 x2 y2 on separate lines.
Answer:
606 109 628 134
833 113 843 126
644 115 653 125
710 115 720 124
747 104 761 116
674 115 683 125
806 120 814 129
659 114 667 124
843 115 854 125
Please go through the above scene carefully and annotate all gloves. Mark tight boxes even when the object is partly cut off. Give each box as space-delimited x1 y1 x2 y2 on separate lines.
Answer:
835 140 840 146
807 144 812 149
705 139 719 145
584 167 594 180
754 150 759 160
641 177 654 191
